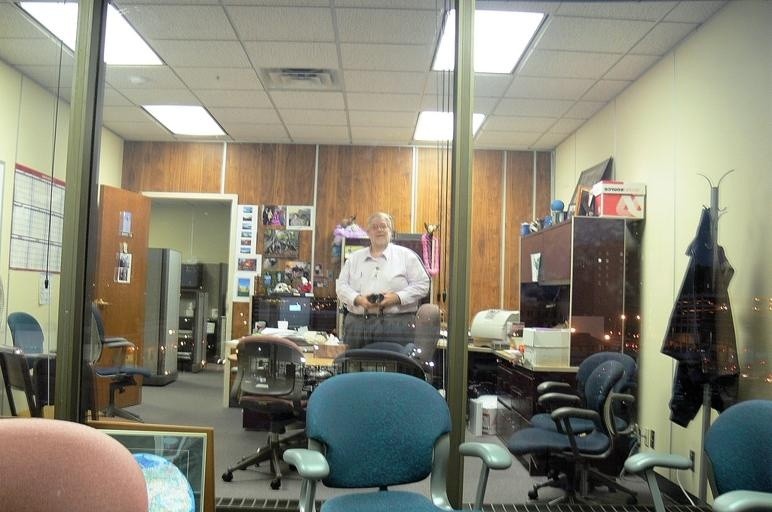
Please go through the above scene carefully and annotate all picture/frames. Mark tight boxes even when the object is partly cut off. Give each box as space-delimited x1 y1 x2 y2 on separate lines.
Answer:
565 158 611 215
232 203 317 303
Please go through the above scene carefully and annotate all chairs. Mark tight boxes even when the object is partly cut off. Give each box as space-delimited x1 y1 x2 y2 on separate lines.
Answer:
7 311 58 406
88 304 153 423
0 414 147 511
0 347 43 417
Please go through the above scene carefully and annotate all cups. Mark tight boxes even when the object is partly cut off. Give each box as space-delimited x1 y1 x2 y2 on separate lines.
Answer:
277 321 288 330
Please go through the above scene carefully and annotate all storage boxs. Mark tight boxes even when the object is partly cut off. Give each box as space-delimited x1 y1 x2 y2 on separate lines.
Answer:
591 182 647 219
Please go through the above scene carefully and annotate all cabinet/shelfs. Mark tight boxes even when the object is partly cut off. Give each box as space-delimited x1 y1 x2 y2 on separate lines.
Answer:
496 216 626 474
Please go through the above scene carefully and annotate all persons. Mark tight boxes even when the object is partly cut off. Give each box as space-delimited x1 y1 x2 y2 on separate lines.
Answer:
335 212 430 350
